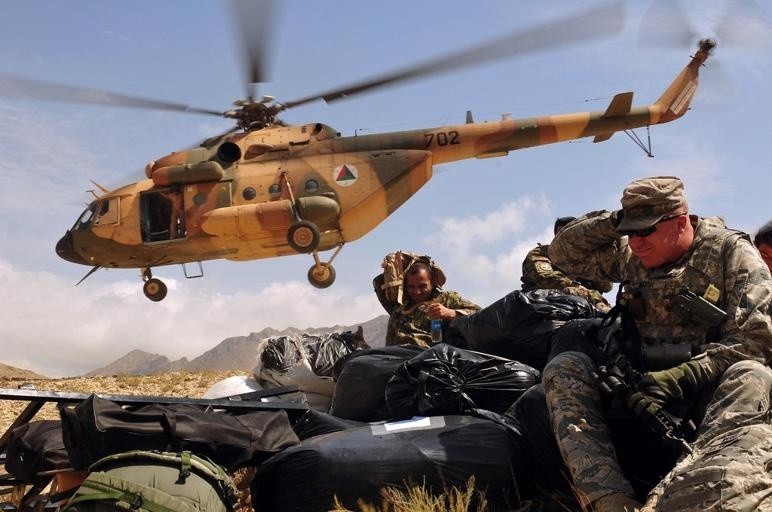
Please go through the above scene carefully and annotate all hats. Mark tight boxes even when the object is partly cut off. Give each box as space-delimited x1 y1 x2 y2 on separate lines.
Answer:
616 175 688 231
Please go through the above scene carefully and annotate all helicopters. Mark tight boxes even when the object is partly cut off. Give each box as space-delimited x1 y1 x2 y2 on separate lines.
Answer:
0 0 771 301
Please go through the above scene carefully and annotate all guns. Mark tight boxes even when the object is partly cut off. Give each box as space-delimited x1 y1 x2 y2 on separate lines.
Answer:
572 306 698 455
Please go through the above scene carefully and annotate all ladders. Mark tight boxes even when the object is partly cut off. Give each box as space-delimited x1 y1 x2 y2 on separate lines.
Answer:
0 384 310 465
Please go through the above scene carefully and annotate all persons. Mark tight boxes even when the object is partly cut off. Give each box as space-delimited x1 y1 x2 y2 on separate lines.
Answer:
373 251 484 347
521 215 612 312
162 189 184 239
753 222 772 274
543 175 772 510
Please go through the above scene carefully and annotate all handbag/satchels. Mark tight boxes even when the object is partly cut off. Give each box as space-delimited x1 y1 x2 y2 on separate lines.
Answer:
4 391 300 512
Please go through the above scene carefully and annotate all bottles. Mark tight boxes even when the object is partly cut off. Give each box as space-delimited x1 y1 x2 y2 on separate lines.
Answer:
430 317 443 343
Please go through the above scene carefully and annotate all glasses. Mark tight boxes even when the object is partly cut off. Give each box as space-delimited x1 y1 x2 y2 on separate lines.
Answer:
626 215 667 238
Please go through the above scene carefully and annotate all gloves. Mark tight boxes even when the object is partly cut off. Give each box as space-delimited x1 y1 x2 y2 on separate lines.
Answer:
629 361 712 420
